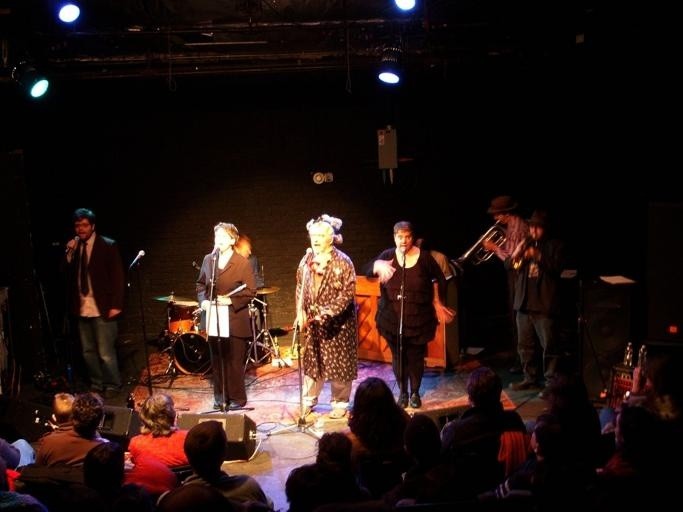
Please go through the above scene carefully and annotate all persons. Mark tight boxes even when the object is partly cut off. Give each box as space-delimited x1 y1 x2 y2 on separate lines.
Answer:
197 222 258 409
286 352 601 510
503 208 571 399
415 236 452 280
66 208 127 400
595 347 682 511
366 219 458 413
2 392 275 510
479 194 529 372
292 214 356 419
230 233 264 290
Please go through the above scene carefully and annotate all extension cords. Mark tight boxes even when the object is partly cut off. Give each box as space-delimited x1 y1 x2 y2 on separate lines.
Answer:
272 359 286 366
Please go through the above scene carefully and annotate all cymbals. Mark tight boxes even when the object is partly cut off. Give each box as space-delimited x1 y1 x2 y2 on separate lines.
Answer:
153 294 194 303
255 285 280 295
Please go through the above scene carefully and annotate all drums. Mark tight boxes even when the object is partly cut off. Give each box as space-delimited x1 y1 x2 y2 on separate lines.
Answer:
167 301 200 335
170 323 212 376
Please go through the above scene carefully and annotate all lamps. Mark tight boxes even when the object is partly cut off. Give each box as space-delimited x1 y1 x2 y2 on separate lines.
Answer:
377 39 404 85
6 55 51 99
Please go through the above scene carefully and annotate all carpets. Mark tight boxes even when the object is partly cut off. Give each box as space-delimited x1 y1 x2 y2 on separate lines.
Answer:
122 351 520 439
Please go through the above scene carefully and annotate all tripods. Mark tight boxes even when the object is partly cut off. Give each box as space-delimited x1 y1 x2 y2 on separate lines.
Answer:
126 264 190 412
199 255 255 415
244 300 276 376
256 295 279 363
29 255 78 401
266 265 326 441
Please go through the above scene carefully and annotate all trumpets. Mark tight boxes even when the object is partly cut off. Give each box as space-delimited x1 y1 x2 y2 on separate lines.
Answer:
450 219 510 272
503 233 537 269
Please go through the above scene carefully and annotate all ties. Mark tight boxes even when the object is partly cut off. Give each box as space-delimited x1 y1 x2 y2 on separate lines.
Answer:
82 242 90 297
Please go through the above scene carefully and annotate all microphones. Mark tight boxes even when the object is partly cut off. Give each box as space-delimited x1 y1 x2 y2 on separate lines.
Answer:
65 235 80 254
304 248 312 264
129 250 145 271
212 246 220 255
401 248 406 267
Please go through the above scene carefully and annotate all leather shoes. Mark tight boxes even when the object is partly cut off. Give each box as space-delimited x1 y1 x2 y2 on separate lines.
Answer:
229 402 242 410
397 395 409 409
213 401 228 409
539 388 552 400
411 396 422 409
508 380 541 392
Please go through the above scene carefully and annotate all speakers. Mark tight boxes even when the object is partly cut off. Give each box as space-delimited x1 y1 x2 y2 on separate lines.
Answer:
177 414 256 460
647 200 683 345
0 396 53 444
414 404 472 432
580 278 630 409
96 406 140 450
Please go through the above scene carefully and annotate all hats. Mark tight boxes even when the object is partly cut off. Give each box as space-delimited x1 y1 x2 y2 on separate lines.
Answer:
525 210 549 224
488 196 518 214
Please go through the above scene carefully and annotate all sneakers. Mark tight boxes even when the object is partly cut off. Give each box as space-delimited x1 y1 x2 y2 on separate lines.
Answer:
299 405 313 423
329 409 348 420
